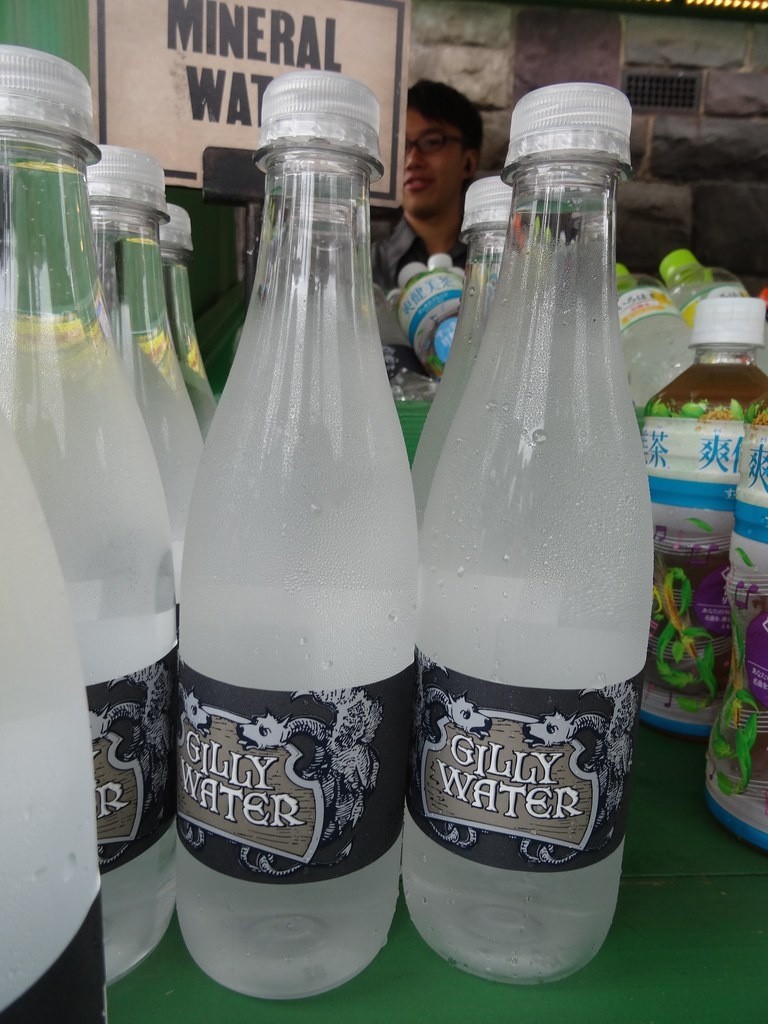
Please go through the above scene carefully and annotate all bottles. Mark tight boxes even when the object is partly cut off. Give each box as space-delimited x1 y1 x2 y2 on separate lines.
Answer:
637 294 768 743
171 68 423 1004
699 398 768 860
426 250 467 285
1 39 182 987
750 285 768 384
610 262 698 413
76 139 209 686
0 412 113 1023
151 197 214 441
410 171 516 530
398 80 659 988
661 247 751 335
398 260 466 380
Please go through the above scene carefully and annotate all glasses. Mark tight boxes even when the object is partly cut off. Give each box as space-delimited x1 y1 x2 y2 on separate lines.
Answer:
404 130 469 155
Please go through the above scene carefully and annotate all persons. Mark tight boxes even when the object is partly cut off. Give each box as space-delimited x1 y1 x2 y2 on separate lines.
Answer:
367 70 490 282
